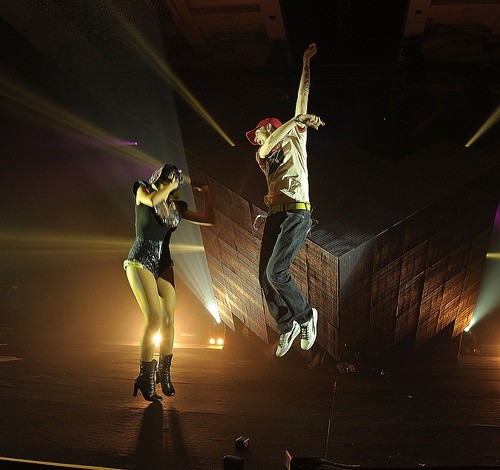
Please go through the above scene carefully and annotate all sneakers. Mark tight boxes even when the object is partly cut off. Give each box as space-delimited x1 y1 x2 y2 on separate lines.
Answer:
275 320 302 357
300 307 318 350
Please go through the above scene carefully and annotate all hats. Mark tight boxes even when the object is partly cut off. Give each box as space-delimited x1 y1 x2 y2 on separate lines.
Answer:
246 117 283 145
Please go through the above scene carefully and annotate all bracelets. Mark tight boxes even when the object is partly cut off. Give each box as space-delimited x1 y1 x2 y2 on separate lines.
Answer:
294 114 304 126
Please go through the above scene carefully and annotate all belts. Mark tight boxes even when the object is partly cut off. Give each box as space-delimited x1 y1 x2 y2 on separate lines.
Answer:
268 203 312 213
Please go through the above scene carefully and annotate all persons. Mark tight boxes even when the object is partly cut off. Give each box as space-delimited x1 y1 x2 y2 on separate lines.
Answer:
124 164 216 401
246 42 325 358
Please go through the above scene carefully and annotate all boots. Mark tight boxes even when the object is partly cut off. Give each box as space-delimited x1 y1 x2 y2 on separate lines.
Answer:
157 353 175 397
132 359 163 402
